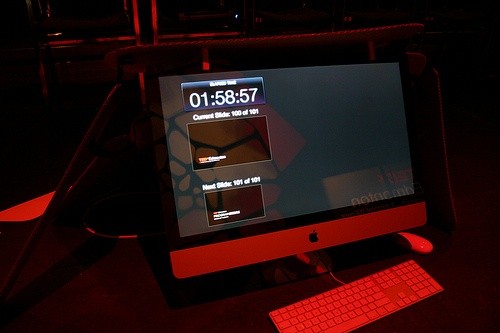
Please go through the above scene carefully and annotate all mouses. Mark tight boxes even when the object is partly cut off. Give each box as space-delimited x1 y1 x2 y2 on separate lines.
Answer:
394 230 433 256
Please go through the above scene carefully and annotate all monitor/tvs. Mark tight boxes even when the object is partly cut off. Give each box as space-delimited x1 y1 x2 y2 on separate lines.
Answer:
135 44 429 284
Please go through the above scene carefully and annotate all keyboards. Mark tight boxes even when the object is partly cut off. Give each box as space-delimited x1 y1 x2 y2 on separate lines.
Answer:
267 258 444 333
